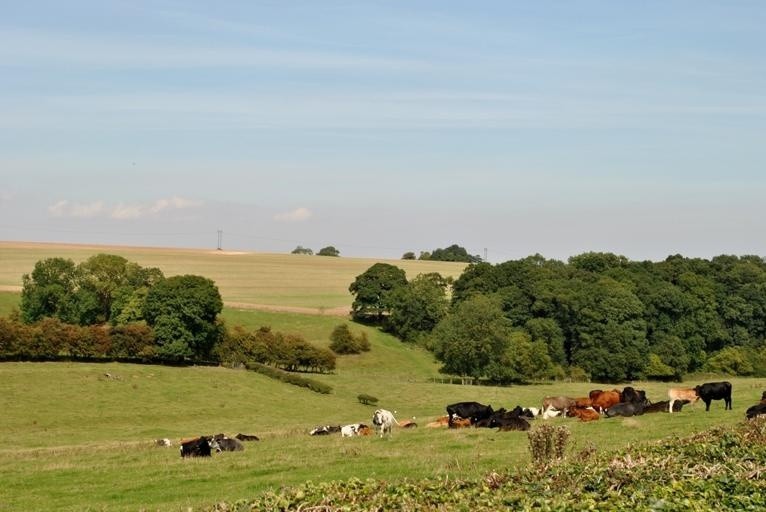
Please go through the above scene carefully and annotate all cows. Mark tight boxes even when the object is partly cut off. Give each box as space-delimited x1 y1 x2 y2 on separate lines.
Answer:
153 438 171 448
746 390 766 420
693 381 733 411
177 432 244 458
425 385 651 433
666 386 700 413
308 408 418 438
235 433 259 442
